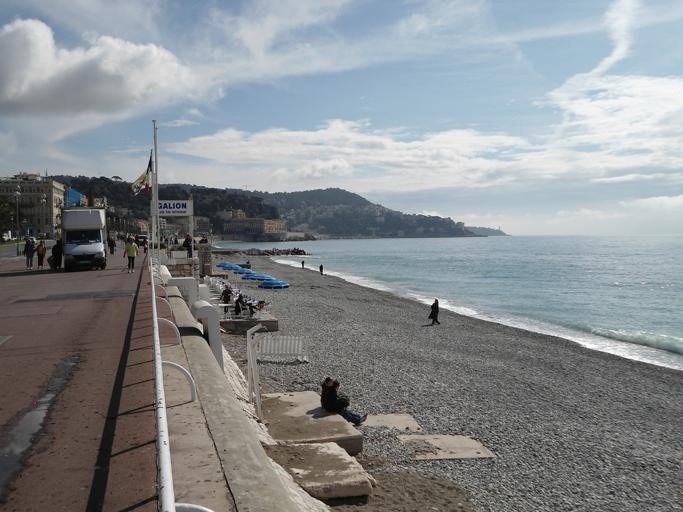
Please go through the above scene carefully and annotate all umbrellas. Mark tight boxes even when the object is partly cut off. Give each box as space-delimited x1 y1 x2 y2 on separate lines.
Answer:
215 260 289 302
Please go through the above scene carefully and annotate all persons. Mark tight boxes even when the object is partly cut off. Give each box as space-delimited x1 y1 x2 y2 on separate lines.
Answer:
320 376 367 426
219 285 232 313
234 294 246 315
21 233 207 273
427 298 439 324
326 380 347 413
319 264 323 276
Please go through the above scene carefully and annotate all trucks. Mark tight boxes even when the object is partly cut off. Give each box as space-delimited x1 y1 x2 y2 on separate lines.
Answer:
61 206 110 271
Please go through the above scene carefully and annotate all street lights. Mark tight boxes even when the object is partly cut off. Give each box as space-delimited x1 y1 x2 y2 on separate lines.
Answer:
54 196 136 242
13 185 21 257
38 193 48 244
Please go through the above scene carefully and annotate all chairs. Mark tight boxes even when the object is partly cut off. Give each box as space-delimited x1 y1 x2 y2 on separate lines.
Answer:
204 274 273 319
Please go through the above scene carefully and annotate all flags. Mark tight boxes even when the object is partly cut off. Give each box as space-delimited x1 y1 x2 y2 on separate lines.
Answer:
129 152 152 196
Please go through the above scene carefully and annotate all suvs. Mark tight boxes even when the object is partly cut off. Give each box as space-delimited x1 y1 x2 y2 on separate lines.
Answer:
135 235 148 245
25 237 36 244
